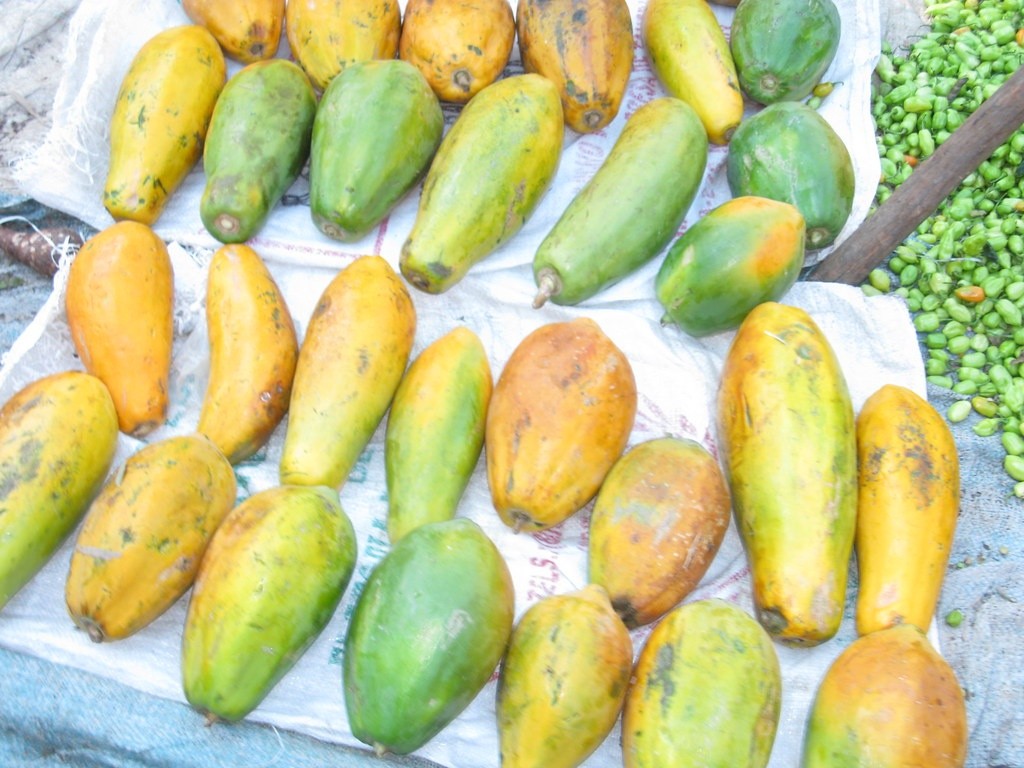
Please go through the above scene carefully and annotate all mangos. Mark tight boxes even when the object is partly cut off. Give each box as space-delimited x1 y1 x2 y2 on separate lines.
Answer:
1 0 968 768
